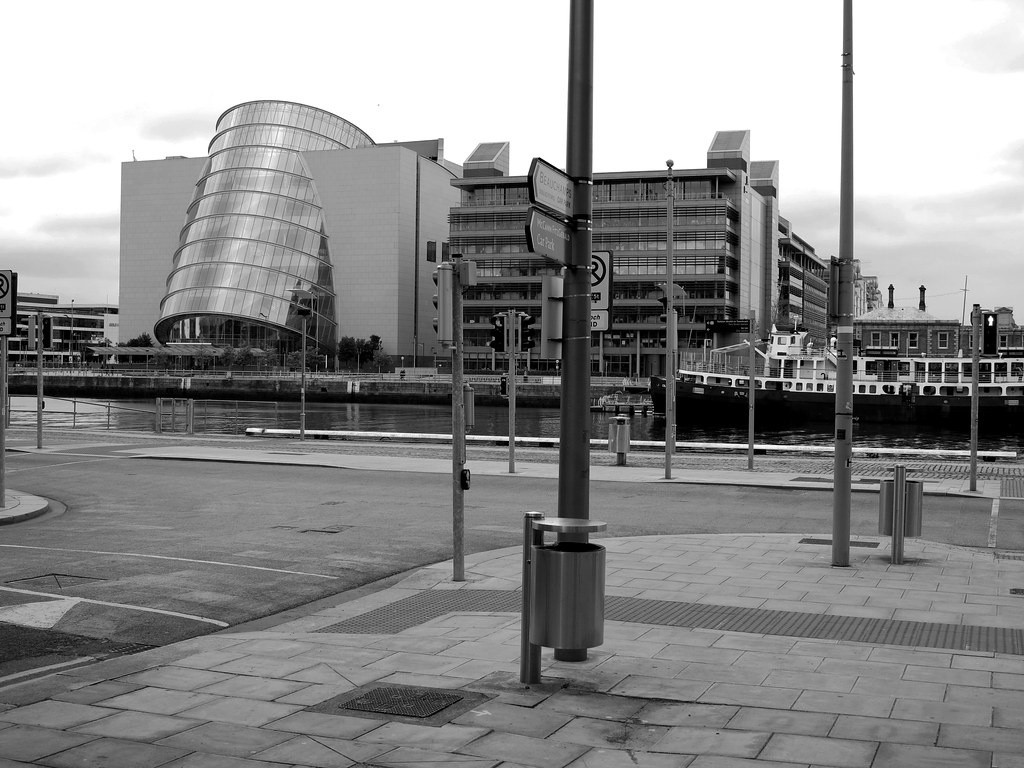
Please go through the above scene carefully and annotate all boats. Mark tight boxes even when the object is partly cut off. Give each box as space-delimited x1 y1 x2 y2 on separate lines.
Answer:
649 283 1024 432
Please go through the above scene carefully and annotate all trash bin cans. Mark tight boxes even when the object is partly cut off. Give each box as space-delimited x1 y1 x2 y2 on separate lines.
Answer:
877 463 924 565
518 510 608 684
608 416 631 465
